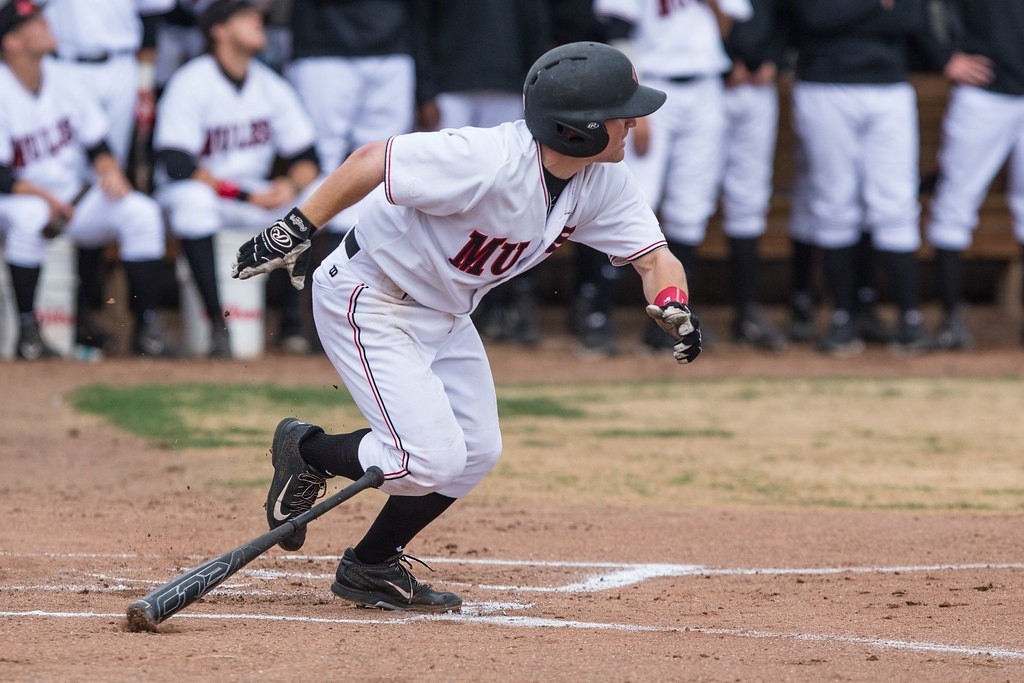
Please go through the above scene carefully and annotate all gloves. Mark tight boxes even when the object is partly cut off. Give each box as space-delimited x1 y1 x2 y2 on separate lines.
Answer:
646 301 702 365
230 206 317 299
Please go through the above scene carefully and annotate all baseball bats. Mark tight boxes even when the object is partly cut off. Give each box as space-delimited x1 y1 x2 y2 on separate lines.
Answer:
125 465 388 632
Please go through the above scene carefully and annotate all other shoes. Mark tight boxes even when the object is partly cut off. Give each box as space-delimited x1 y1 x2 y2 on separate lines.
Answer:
485 279 542 348
569 284 620 346
205 316 233 359
74 325 119 355
15 316 59 360
130 301 180 356
280 315 307 354
728 283 975 358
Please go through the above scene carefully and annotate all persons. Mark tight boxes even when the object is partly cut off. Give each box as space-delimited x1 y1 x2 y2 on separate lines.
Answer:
234 41 704 613
0 0 1024 363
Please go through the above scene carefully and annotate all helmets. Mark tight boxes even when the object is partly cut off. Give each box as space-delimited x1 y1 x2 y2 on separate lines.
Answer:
522 42 668 157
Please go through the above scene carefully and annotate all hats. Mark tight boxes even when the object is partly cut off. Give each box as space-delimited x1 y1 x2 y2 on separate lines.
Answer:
1 0 50 38
200 1 254 30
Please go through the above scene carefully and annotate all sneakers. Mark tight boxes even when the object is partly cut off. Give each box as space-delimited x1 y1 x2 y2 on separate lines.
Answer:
328 546 463 612
266 416 328 551
